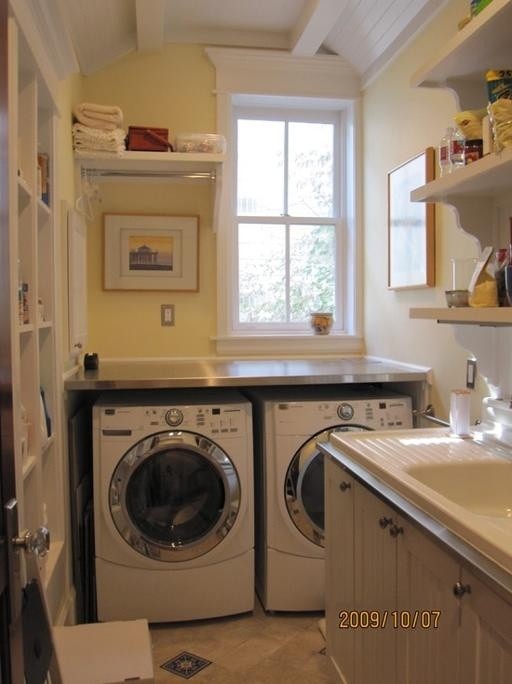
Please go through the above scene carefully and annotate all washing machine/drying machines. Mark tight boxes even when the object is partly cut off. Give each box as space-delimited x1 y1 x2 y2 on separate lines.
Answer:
260 392 413 617
88 395 259 622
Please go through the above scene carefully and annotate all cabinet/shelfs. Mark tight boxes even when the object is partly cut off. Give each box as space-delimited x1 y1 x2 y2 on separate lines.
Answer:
458 567 512 684
350 476 461 683
407 0 512 328
4 12 71 684
320 457 352 683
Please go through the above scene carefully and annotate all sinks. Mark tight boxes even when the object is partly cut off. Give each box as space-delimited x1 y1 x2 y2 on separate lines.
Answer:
402 455 512 526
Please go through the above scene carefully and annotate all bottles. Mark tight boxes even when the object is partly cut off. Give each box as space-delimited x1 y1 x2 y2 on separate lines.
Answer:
439 123 465 178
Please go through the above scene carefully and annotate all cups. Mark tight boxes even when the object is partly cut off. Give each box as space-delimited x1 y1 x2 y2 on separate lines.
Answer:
444 256 478 308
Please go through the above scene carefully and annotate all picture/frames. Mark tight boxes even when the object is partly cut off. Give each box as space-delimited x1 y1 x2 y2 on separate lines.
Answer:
385 146 437 291
101 212 200 292
